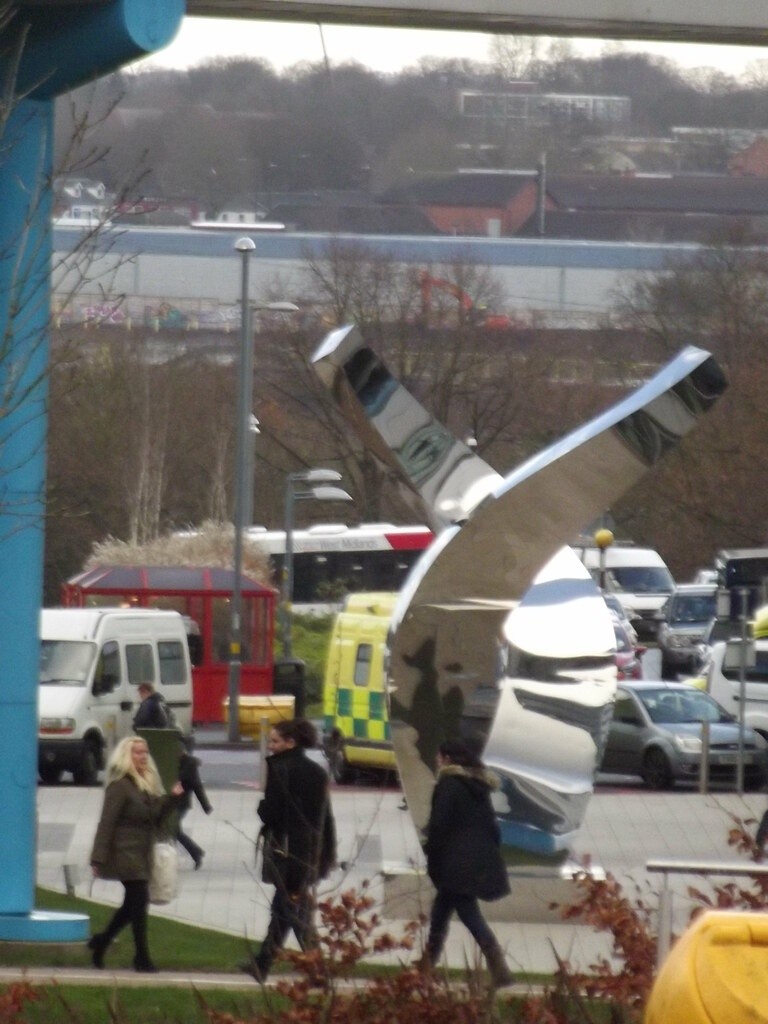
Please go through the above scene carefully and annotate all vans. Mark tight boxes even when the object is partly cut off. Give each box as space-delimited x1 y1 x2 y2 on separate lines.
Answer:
37 608 196 786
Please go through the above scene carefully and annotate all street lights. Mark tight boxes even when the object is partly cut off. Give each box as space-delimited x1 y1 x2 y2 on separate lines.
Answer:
228 238 299 744
594 529 614 597
282 468 353 658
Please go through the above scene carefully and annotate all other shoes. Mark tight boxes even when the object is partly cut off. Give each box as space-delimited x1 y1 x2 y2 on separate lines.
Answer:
133 954 159 972
294 976 325 992
195 850 205 870
244 960 270 984
87 932 113 969
483 975 517 991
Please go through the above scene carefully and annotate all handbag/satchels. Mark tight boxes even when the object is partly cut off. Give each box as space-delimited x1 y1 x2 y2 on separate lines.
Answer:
470 841 511 902
149 842 177 904
263 820 313 894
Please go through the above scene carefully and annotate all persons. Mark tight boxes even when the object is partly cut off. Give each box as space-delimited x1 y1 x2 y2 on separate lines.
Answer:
168 736 214 872
236 718 337 988
86 736 184 973
411 736 517 992
132 682 178 735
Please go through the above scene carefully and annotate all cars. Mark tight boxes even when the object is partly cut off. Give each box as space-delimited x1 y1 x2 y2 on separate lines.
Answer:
596 680 768 791
572 529 768 738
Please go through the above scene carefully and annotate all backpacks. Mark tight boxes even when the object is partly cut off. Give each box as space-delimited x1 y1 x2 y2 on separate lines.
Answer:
147 697 176 728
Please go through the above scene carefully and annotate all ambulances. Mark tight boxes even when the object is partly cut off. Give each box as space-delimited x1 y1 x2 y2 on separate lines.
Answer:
322 591 403 785
